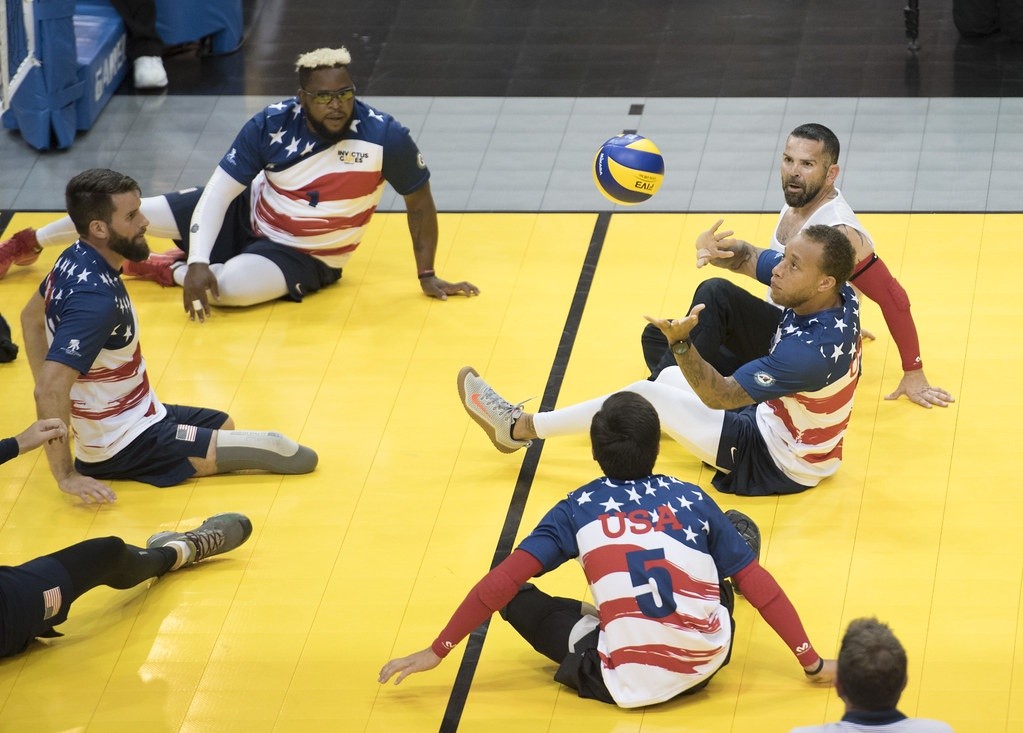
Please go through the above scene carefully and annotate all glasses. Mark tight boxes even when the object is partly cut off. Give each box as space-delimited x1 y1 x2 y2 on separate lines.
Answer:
303 83 358 104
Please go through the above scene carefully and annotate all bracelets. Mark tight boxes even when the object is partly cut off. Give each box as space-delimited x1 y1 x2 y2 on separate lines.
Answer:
417 271 435 278
803 657 824 675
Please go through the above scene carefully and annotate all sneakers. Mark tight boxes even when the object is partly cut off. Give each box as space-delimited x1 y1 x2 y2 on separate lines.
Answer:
723 509 761 595
0 228 43 279
147 512 252 566
457 366 537 454
121 248 187 287
133 56 169 88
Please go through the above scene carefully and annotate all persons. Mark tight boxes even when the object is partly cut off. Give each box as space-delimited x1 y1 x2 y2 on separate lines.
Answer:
641 122 956 408
20 169 318 506
376 390 840 710
792 619 954 733
457 219 865 496
108 0 168 89
0 418 254 658
1 47 481 323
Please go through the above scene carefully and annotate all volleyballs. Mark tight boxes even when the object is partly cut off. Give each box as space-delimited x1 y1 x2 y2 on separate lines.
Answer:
591 133 668 207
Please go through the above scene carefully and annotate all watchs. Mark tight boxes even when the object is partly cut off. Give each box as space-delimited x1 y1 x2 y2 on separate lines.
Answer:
667 337 692 354
